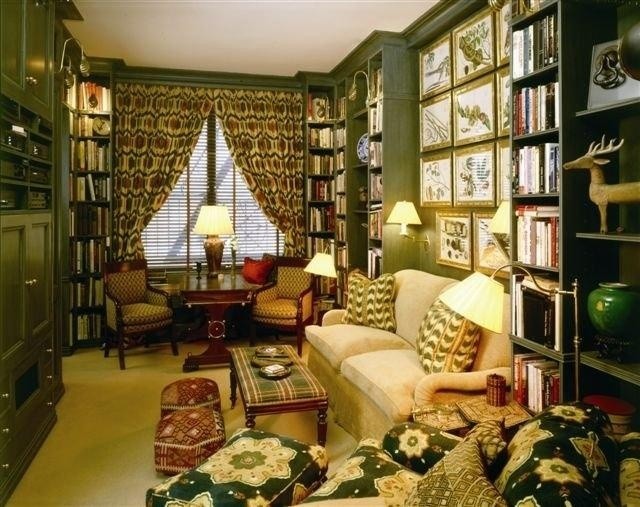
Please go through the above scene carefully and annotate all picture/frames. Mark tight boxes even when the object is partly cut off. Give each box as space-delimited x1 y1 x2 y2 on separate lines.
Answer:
452 142 495 208
419 28 452 102
419 90 453 153
496 1 516 67
497 138 511 208
496 66 513 137
419 152 452 208
451 5 496 87
452 73 496 147
473 212 510 280
436 209 472 271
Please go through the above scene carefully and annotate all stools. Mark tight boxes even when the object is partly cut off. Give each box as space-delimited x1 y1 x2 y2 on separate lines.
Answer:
155 409 226 477
161 378 222 415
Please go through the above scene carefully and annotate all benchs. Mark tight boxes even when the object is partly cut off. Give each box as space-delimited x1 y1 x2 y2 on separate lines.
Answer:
145 428 329 507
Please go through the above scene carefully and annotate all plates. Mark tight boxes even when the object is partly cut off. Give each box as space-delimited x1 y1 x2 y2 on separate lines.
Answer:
356 132 367 163
255 344 285 354
259 365 291 380
252 355 292 367
617 22 639 81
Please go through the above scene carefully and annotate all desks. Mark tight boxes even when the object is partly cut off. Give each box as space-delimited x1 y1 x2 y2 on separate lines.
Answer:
180 274 257 372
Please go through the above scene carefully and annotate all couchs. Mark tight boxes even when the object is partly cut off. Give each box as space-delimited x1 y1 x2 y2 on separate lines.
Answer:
304 269 511 444
101 259 179 370
294 399 640 507
253 255 319 358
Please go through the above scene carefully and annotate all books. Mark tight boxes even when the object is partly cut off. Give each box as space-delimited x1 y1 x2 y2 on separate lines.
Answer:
368 202 382 238
71 237 110 274
510 81 560 137
68 203 110 237
307 177 336 201
308 92 347 120
68 313 105 347
336 193 346 215
307 235 335 259
514 203 560 270
308 151 344 175
368 99 382 136
68 277 105 311
367 247 384 280
65 74 111 111
313 274 337 327
368 140 383 168
370 172 383 200
511 142 560 196
70 138 111 172
369 67 384 102
336 171 346 194
69 173 111 202
337 243 348 268
513 352 560 415
307 126 346 149
511 14 559 79
336 216 347 242
336 270 349 308
309 204 336 232
511 272 560 353
69 111 95 137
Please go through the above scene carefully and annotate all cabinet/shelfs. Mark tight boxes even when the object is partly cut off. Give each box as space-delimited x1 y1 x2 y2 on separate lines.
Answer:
0 212 56 504
1 94 55 214
336 79 369 272
306 82 336 267
559 1 640 441
346 66 370 274
68 69 113 353
511 1 562 417
0 0 54 122
369 50 420 280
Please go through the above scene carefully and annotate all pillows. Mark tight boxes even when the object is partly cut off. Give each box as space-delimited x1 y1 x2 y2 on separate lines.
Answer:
404 432 510 507
415 298 482 376
342 272 398 334
467 415 507 466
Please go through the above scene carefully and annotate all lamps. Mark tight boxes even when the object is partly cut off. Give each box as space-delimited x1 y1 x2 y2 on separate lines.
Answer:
386 200 422 236
193 205 235 278
490 199 512 234
439 266 580 402
304 252 337 301
